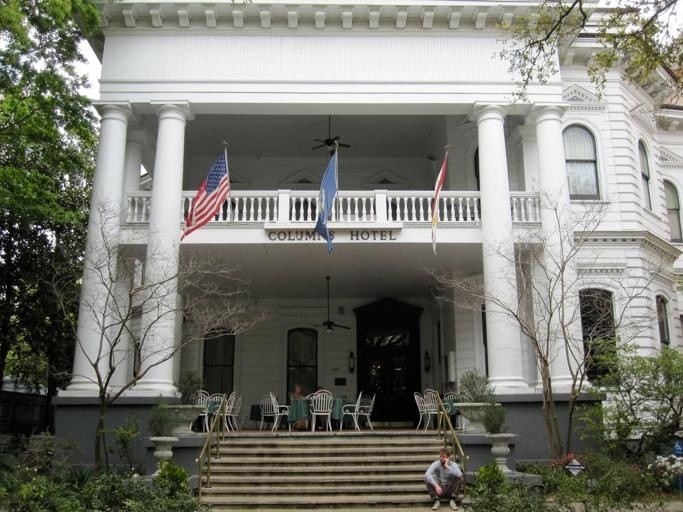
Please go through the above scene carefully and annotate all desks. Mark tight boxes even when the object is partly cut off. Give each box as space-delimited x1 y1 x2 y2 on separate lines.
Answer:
251 404 287 431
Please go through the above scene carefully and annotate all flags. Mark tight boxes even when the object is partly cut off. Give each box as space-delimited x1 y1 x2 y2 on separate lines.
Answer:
179 140 231 243
312 139 339 255
431 151 448 256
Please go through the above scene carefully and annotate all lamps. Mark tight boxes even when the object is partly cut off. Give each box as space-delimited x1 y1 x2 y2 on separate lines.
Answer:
326 325 333 333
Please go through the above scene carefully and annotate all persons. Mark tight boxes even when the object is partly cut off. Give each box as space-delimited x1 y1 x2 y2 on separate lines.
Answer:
291 382 308 429
312 385 329 430
423 447 464 511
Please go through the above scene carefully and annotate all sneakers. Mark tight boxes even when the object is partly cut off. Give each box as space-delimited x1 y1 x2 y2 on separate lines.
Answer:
449 500 458 511
432 500 441 510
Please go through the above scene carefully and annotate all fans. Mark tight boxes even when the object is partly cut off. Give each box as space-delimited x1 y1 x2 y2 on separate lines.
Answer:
312 277 351 334
312 115 351 151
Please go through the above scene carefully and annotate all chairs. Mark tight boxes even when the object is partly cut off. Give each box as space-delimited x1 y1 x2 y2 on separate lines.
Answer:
193 390 244 432
260 389 376 432
414 388 458 431
